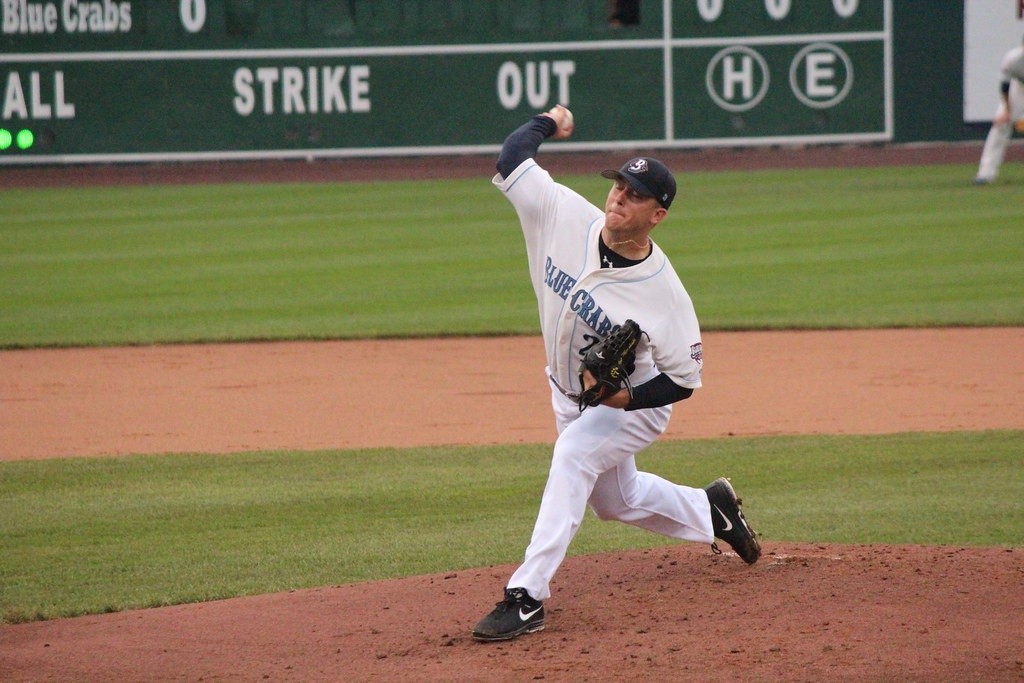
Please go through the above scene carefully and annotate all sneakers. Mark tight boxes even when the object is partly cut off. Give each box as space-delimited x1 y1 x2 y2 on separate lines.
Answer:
704 477 762 564
473 587 544 641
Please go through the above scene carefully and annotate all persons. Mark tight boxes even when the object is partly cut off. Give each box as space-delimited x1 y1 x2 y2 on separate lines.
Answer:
470 105 762 641
973 32 1024 184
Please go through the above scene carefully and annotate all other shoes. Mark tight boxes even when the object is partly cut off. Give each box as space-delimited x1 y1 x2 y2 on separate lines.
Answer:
975 179 992 185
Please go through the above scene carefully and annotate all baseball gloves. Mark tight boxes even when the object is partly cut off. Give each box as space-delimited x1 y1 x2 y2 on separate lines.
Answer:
579 319 652 413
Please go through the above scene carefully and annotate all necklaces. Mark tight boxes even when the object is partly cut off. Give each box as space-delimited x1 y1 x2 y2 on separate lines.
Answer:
608 238 649 249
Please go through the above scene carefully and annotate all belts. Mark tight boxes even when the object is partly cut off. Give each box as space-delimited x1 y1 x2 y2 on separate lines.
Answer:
550 375 580 404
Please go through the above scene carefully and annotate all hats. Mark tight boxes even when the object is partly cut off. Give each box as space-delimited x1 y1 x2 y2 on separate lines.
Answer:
601 157 676 210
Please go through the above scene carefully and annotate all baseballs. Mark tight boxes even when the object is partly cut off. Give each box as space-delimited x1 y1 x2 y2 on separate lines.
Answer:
551 107 574 130
